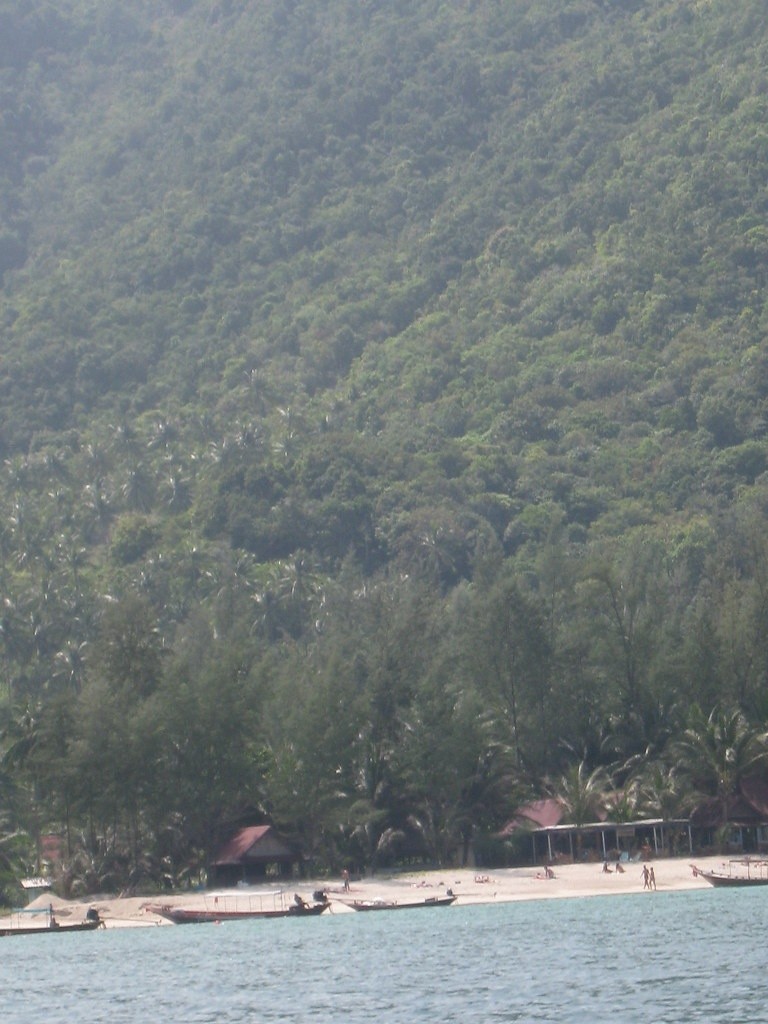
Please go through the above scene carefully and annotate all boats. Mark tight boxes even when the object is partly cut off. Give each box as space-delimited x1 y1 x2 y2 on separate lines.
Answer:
0 903 106 937
138 886 335 924
691 857 768 889
341 893 457 912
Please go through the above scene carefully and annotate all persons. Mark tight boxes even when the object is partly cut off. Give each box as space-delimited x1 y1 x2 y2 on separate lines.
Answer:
640 866 649 889
544 866 555 878
616 864 624 873
341 867 350 890
649 868 656 889
602 862 608 873
49 918 59 927
